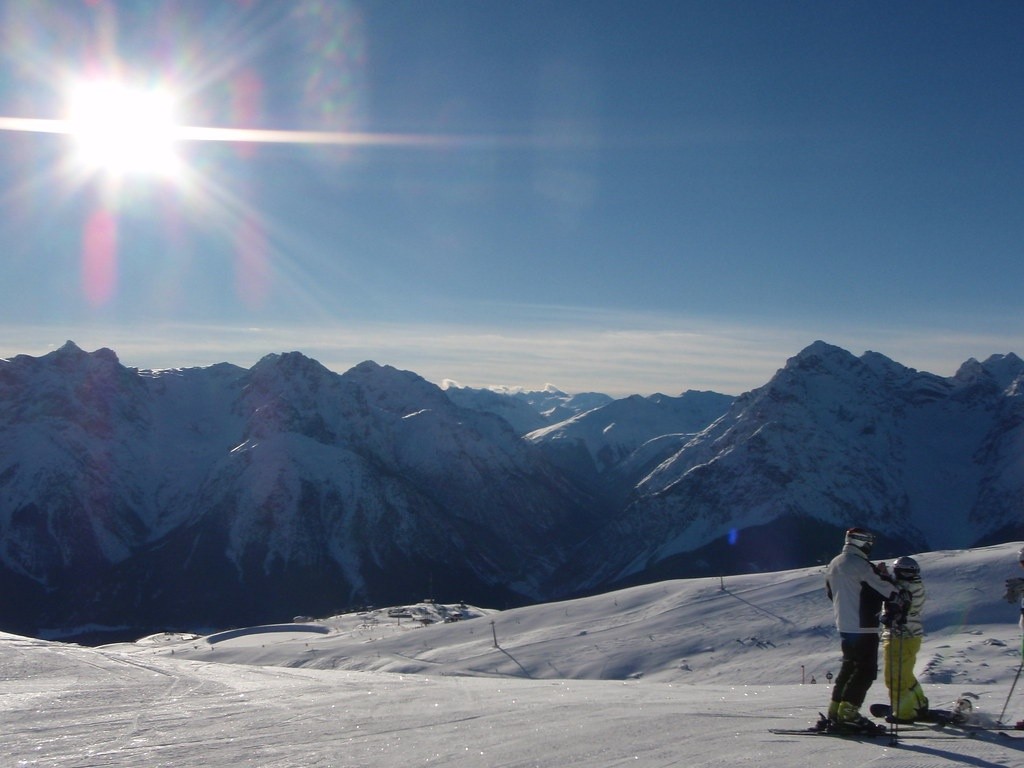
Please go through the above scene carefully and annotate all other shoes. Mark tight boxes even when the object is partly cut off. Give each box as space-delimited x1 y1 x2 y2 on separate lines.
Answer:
885 707 929 724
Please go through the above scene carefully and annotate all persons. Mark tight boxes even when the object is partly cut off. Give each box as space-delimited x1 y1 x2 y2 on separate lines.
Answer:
879 555 929 726
824 525 912 732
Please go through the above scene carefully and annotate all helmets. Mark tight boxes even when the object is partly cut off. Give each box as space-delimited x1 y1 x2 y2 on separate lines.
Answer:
845 527 875 554
893 556 920 572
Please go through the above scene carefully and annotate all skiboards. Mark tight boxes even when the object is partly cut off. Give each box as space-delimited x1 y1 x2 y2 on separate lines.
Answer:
766 726 900 739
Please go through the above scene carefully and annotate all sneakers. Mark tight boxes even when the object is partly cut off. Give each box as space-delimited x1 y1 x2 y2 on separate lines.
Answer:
828 702 877 727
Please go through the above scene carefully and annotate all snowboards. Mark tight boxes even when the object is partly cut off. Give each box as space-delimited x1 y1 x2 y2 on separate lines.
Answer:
869 703 968 725
